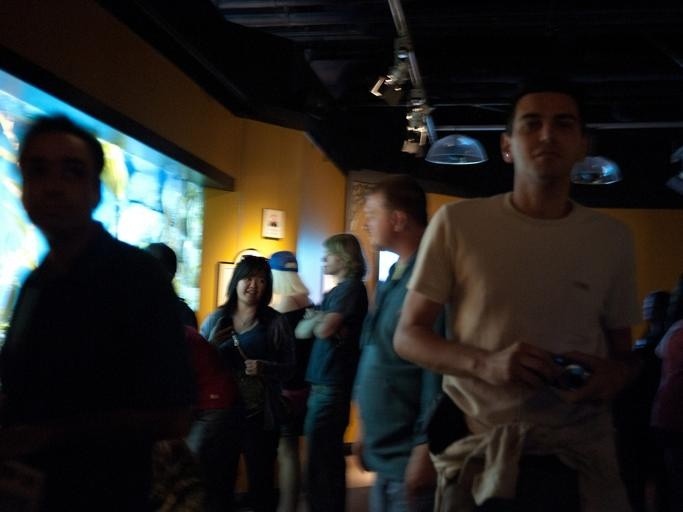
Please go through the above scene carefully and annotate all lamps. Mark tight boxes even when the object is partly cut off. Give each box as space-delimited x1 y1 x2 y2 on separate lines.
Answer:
569 126 620 185
370 36 437 160
424 125 488 165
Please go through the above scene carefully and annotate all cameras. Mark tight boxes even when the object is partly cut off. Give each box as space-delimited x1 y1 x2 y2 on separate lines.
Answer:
551 353 591 390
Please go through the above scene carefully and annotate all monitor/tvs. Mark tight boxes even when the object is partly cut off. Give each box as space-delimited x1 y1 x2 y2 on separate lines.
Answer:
378 249 400 283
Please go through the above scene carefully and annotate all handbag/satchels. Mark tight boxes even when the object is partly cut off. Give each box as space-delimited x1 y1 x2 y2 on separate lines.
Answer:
277 389 307 433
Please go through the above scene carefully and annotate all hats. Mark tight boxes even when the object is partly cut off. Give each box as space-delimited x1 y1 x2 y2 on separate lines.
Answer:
267 251 298 271
